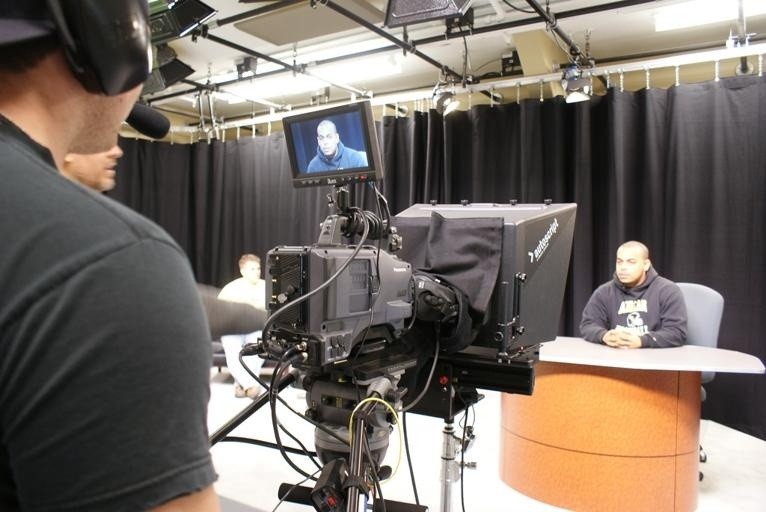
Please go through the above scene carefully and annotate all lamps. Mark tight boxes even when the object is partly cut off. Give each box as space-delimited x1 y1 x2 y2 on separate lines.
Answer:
425 63 469 121
138 0 257 99
383 0 475 37
558 28 604 104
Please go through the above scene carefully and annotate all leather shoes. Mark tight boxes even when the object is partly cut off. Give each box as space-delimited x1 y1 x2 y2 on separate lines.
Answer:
247 389 257 399
236 386 245 397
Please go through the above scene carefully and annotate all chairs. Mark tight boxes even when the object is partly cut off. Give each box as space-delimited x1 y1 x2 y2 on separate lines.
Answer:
647 277 727 484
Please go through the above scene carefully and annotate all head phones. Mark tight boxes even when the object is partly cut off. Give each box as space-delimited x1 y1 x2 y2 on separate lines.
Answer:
0 0 153 97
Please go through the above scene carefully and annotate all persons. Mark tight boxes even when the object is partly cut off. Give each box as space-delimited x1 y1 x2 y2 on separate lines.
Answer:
60 145 125 196
305 120 368 175
216 255 272 399
577 240 690 350
0 0 224 511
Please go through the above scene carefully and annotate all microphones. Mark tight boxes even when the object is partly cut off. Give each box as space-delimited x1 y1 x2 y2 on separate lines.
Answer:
125 102 170 139
